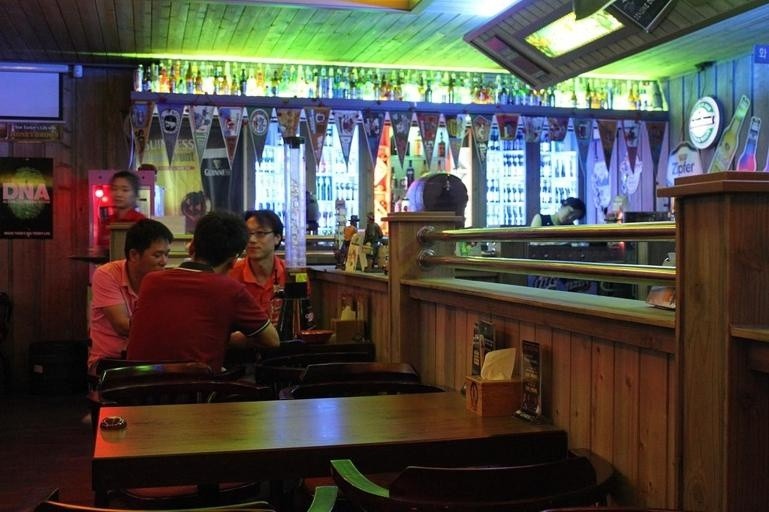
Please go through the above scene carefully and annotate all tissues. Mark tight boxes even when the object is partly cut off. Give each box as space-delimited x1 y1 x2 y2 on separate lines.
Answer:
329 305 365 344
464 348 523 418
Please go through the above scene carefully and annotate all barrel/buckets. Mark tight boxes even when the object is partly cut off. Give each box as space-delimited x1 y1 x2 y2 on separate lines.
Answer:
407 173 468 211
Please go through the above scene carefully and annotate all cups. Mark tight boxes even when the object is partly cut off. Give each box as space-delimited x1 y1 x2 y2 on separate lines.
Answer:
457 240 497 258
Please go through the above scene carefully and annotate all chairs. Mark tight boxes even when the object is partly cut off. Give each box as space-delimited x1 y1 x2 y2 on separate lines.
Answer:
62 325 446 429
35 467 283 512
297 447 620 512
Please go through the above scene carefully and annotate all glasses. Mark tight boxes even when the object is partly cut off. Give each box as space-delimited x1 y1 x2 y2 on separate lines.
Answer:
250 228 276 238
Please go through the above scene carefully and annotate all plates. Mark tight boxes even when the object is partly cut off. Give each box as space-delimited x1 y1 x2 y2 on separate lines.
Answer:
71 254 109 265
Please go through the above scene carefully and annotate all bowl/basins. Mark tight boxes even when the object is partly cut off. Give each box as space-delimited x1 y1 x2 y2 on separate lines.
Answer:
297 329 334 344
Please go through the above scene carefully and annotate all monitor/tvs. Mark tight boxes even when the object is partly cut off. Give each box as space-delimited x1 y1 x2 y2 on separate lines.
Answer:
623 211 670 251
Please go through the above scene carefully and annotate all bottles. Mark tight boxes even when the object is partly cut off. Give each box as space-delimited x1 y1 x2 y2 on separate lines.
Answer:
737 116 760 173
255 126 288 238
484 123 581 226
314 120 359 234
386 122 450 212
708 95 750 174
132 58 666 114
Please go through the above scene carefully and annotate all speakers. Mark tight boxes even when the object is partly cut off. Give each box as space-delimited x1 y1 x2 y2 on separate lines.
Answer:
73 65 83 78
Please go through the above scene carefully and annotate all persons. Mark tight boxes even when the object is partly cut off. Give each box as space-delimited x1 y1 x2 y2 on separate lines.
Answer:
94 171 146 262
86 216 172 400
223 209 312 329
123 209 281 373
529 196 587 228
334 212 382 272
